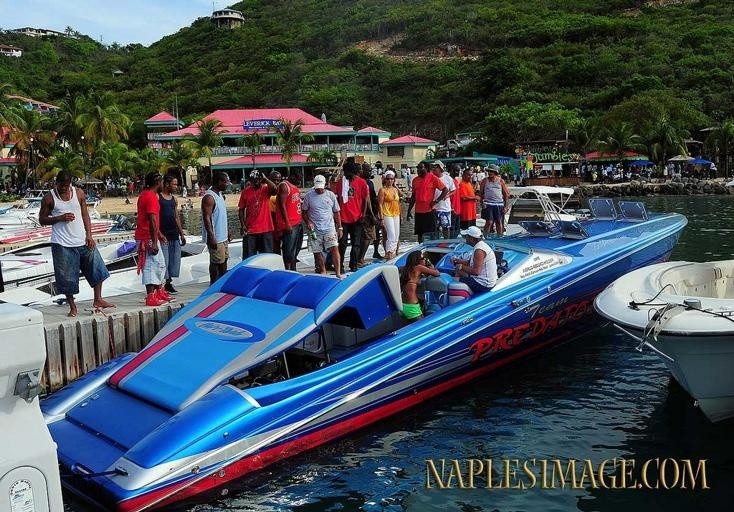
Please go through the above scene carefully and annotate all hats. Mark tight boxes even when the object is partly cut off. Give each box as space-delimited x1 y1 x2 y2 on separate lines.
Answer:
485 164 500 173
249 169 262 181
460 226 482 238
383 170 396 177
431 160 445 171
314 175 326 189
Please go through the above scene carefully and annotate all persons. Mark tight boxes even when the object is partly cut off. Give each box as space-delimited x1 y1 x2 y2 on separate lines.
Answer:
371 167 377 176
517 161 718 187
381 167 407 254
195 180 200 197
401 165 407 177
406 172 412 191
276 167 303 271
238 169 277 261
72 175 144 199
182 185 187 198
355 163 384 267
201 172 233 286
460 163 510 181
377 170 403 259
198 182 205 196
513 172 518 185
240 178 245 190
134 171 176 306
458 169 481 230
406 161 448 244
158 175 186 295
378 167 382 174
0 171 56 197
406 167 411 175
39 170 116 317
302 174 343 279
430 160 457 239
332 162 368 272
480 163 510 235
450 226 497 293
0 260 7 304
187 199 194 208
400 250 440 320
267 171 282 253
448 164 460 239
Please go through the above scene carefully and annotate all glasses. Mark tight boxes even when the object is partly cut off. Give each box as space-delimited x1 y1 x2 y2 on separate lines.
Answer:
386 177 396 181
419 257 425 261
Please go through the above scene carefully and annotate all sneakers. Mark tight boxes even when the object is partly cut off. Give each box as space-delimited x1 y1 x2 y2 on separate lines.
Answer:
156 288 176 302
164 284 178 294
146 293 167 305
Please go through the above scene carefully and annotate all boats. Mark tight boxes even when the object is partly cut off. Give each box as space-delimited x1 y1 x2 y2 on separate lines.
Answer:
592 257 734 420
0 208 117 249
0 232 385 307
40 200 687 512
474 185 591 238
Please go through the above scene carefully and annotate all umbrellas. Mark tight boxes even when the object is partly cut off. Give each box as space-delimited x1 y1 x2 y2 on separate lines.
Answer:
668 154 696 161
578 150 649 161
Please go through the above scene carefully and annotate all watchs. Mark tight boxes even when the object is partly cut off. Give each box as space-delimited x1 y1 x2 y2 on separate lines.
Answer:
338 227 344 230
458 264 462 270
